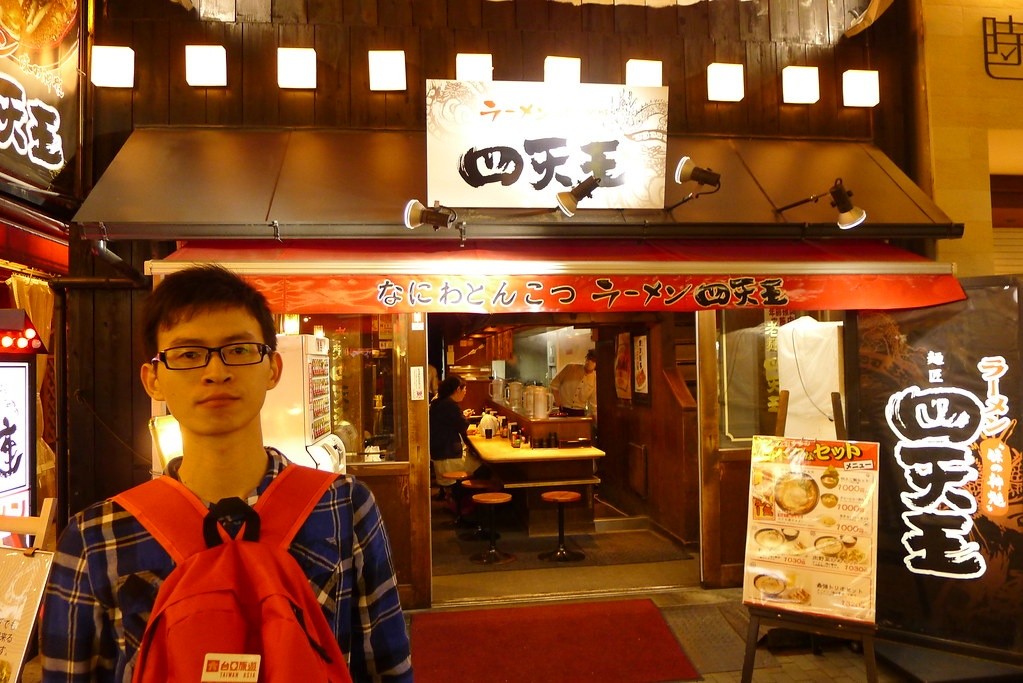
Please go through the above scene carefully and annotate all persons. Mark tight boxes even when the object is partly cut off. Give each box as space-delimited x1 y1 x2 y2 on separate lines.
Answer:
428 364 492 525
39 262 413 683
551 348 598 428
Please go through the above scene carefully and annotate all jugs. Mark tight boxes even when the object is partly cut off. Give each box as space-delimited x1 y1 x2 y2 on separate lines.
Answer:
488 379 553 420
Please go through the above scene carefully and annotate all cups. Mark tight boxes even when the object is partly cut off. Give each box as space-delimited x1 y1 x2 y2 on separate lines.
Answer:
485 428 492 439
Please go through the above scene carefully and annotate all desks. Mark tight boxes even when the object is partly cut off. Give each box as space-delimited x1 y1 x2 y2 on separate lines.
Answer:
466 428 606 539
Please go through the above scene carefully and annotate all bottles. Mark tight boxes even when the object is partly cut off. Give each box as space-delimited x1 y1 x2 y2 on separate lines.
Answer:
310 359 331 439
482 407 520 448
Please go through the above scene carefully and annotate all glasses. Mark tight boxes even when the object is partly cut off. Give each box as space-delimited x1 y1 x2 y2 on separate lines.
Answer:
150 341 273 370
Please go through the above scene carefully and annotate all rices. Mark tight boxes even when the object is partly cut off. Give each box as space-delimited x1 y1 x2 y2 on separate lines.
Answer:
783 528 798 535
841 535 855 542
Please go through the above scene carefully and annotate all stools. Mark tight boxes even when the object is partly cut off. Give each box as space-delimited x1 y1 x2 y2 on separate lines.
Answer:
458 478 500 541
442 470 473 531
538 490 585 562
469 491 517 565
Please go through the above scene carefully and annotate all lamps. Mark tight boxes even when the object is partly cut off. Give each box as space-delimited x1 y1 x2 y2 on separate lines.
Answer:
405 199 467 248
666 156 722 213
773 178 867 230
552 176 601 217
89 43 881 108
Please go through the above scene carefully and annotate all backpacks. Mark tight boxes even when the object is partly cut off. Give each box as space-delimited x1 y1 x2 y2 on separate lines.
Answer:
107 465 352 682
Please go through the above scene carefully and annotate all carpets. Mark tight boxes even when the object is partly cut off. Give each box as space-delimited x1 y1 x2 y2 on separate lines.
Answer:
411 598 704 683
